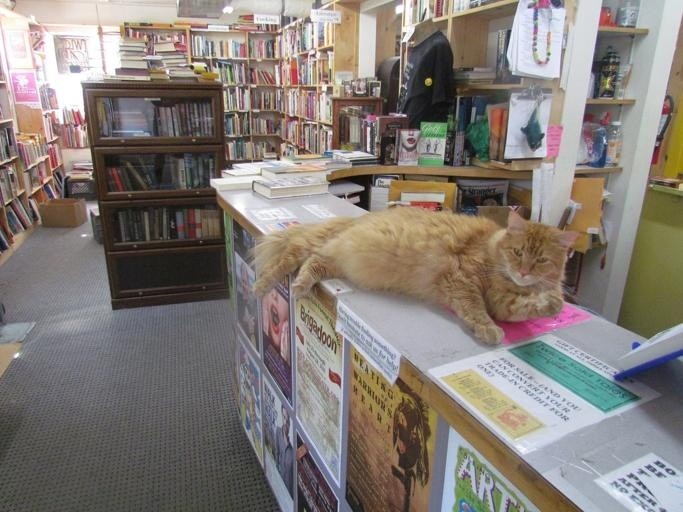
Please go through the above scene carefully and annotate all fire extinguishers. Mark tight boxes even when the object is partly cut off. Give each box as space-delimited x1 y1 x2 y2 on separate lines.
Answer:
651 95 674 164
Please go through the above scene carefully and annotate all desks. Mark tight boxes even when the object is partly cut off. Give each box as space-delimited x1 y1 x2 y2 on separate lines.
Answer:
330 94 385 152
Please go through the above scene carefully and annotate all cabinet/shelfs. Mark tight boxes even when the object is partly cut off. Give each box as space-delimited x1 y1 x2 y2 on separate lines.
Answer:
0 8 66 266
393 1 682 327
81 77 230 311
119 1 357 157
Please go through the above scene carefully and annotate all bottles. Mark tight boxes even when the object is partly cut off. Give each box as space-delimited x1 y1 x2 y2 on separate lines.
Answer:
597 49 621 99
602 119 624 169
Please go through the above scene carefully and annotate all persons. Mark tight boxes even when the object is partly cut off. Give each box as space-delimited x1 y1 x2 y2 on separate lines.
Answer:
237 263 256 349
276 405 293 499
263 288 290 361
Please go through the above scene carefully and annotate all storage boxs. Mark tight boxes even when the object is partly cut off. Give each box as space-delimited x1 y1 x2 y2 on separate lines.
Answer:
38 198 86 228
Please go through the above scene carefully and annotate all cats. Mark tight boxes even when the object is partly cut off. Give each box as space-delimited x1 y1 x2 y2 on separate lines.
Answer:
245 203 582 347
